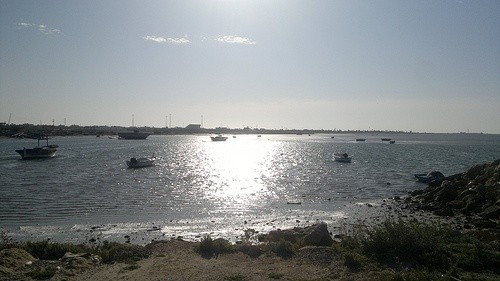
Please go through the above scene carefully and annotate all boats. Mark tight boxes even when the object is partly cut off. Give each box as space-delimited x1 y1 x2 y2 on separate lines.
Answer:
332 152 351 163
15 137 57 159
126 152 158 168
211 136 228 141
117 131 152 139
